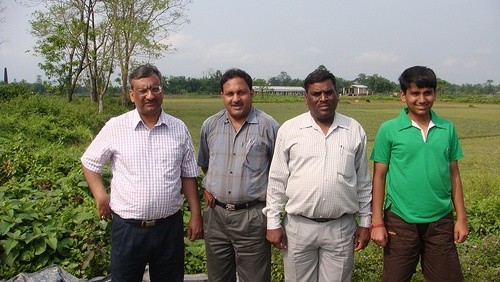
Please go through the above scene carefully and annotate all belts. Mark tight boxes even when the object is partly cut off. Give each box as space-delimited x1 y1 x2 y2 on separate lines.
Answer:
297 213 347 223
112 209 182 229
214 199 262 211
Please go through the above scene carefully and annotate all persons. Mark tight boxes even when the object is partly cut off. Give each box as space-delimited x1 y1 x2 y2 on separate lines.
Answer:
371 65 468 282
197 69 281 282
81 64 205 282
262 70 372 282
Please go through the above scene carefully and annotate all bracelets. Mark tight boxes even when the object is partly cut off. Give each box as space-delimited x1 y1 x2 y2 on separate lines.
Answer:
372 224 385 227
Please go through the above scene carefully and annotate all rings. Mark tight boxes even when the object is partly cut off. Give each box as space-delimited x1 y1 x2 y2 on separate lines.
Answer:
102 215 105 218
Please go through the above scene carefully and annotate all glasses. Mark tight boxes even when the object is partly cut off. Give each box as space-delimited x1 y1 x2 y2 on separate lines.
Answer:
132 86 163 96
